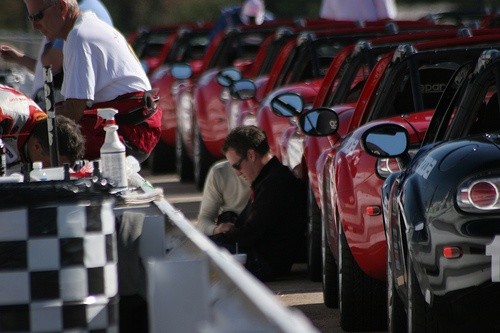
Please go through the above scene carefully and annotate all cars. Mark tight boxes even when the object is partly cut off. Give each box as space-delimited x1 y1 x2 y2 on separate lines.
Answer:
123 18 500 332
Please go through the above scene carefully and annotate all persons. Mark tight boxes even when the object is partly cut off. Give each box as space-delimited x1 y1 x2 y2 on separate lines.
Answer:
0 84 85 173
24 0 162 161
196 161 252 236
0 35 50 96
32 0 113 104
209 125 309 283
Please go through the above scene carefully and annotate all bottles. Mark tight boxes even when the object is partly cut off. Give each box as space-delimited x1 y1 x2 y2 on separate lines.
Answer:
32 161 48 181
0 139 7 177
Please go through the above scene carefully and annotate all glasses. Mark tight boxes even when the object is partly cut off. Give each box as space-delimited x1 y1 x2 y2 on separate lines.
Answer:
29 5 51 22
232 157 244 170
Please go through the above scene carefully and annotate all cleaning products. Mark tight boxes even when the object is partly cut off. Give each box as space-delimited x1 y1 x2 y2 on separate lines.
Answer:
83 108 127 188
